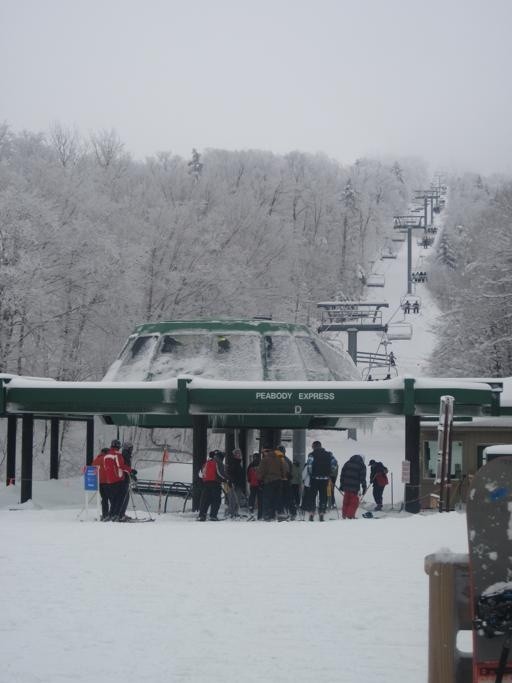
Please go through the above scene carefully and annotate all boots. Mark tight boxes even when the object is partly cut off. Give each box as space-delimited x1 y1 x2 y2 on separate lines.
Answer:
309 514 324 521
374 504 383 511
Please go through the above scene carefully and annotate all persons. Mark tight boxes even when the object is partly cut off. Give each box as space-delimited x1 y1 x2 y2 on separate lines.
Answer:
92 447 111 518
404 300 411 313
120 441 137 520
195 441 389 520
413 300 419 313
102 439 132 520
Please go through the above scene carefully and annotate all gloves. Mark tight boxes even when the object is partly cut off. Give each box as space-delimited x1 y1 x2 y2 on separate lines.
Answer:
130 469 137 482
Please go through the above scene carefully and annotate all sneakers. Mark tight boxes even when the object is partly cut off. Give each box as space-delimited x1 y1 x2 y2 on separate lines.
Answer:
197 515 218 521
101 513 131 521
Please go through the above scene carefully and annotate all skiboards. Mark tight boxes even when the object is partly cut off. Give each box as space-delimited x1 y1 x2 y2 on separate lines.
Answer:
189 498 343 522
80 518 154 522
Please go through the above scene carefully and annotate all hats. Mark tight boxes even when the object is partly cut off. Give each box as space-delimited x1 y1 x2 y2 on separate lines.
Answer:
368 460 375 465
111 440 122 448
209 441 321 459
